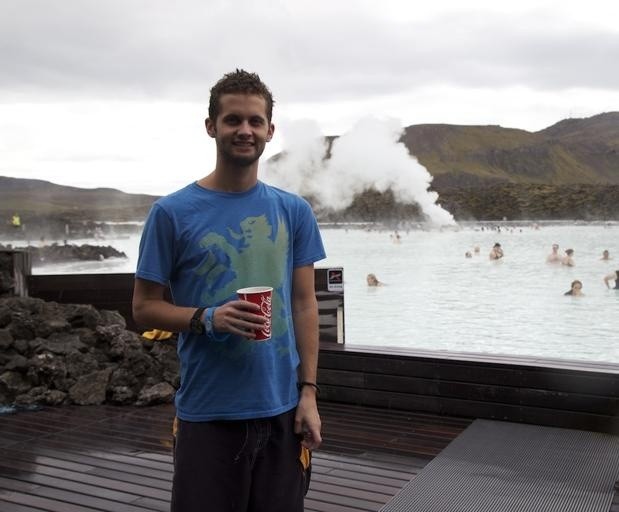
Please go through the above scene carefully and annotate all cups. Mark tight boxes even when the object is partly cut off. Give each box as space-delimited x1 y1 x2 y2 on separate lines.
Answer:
235 285 273 343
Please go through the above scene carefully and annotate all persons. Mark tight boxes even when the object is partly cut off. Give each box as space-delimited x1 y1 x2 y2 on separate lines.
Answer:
601 250 614 260
367 274 386 287
561 249 575 267
344 226 539 259
132 67 327 512
603 270 619 289
564 280 585 296
546 244 563 265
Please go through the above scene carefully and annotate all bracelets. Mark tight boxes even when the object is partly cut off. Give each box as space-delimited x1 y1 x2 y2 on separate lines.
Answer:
205 306 232 343
296 381 322 393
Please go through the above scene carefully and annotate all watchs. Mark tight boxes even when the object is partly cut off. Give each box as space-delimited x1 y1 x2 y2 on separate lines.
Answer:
189 305 210 336
